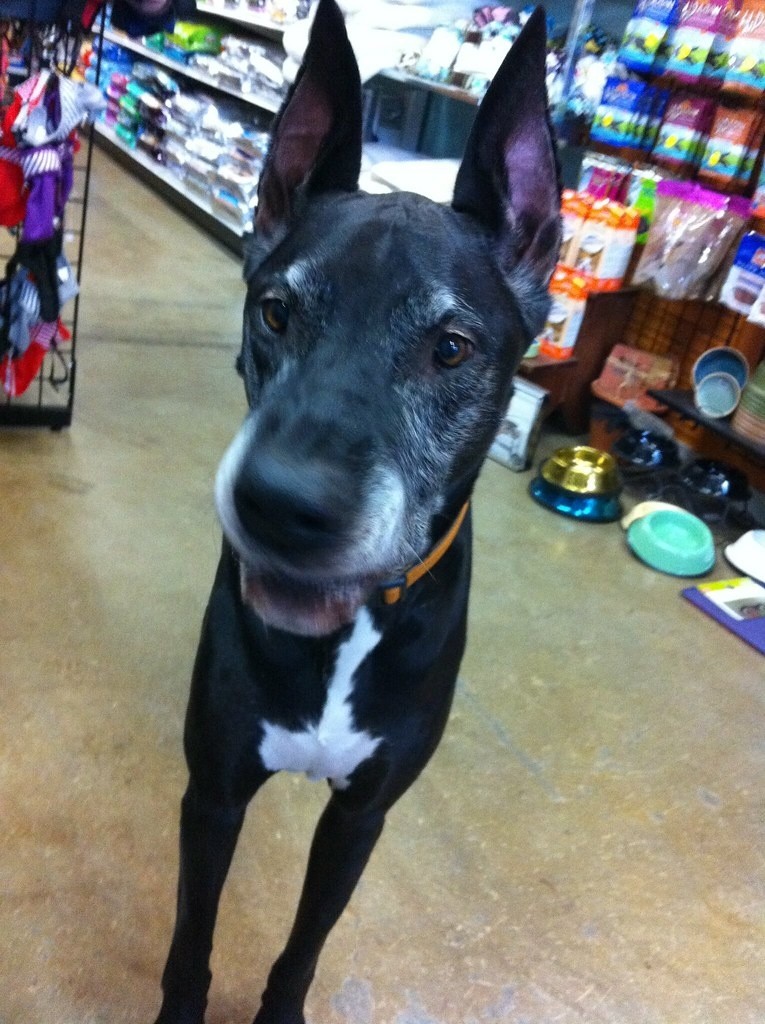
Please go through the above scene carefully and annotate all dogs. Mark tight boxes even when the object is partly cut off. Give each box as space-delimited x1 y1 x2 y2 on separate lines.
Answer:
154 0 563 1024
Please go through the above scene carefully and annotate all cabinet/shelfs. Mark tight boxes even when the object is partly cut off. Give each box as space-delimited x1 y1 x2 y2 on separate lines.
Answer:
0 1 765 464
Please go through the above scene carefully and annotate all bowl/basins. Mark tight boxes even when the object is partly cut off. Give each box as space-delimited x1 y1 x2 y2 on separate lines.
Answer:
625 509 717 577
724 530 765 585
528 477 624 524
538 444 625 497
690 347 765 445
621 500 683 533
